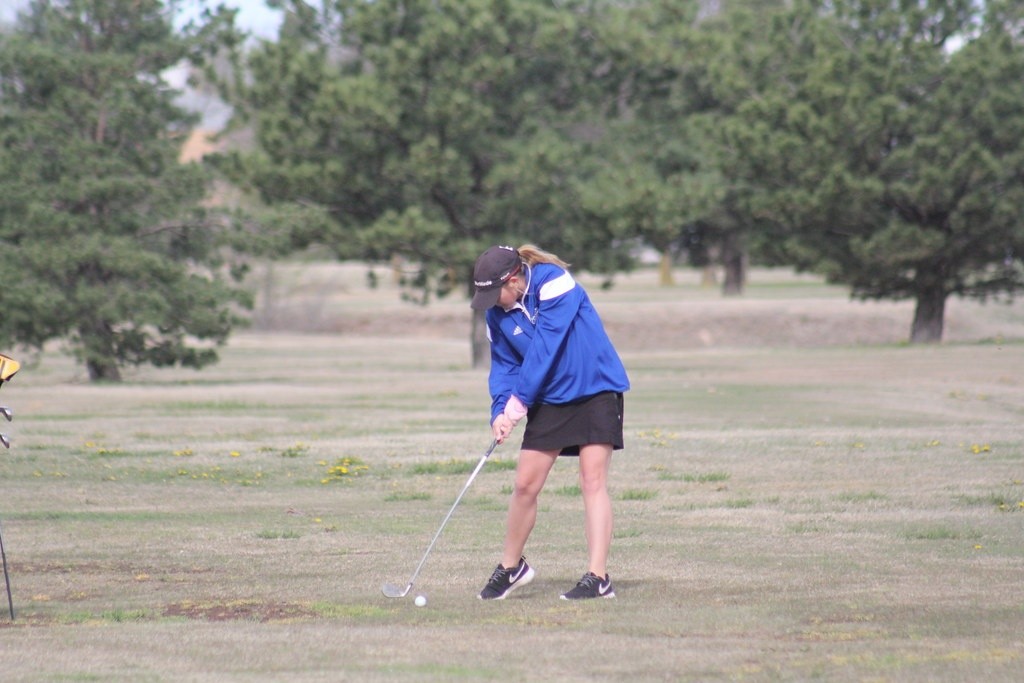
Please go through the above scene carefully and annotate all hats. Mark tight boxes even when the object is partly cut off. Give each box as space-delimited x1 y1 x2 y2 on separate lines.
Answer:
470 246 522 310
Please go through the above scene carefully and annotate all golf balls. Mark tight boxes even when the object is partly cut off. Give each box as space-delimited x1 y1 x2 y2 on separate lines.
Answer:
414 596 426 606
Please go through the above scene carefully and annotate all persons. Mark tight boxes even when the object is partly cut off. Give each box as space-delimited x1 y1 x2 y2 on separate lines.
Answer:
470 244 630 601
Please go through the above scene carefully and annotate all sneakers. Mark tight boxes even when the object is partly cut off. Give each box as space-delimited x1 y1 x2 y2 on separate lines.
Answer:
477 556 535 601
560 571 615 600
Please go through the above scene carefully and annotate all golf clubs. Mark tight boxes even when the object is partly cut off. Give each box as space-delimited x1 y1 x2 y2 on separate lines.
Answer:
380 440 498 598
